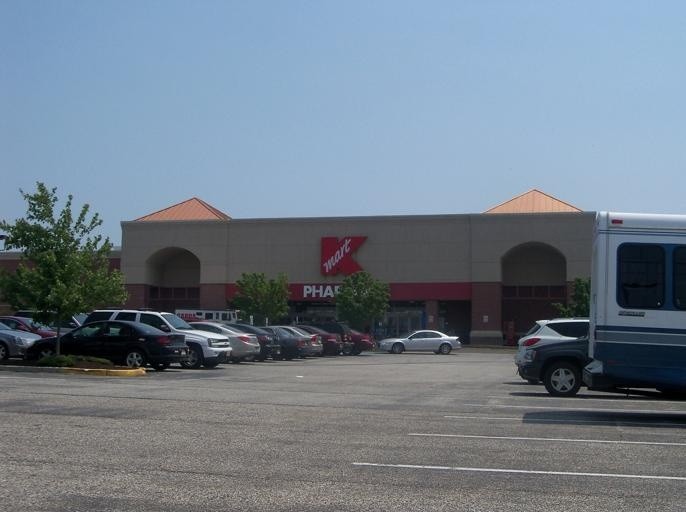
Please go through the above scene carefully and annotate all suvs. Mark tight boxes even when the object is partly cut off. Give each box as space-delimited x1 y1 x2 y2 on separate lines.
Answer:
514 316 589 368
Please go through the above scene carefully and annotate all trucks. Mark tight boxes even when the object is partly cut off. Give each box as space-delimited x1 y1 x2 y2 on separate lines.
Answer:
582 211 686 393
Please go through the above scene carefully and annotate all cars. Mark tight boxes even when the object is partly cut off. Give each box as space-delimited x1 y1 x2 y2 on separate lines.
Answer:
377 329 464 355
518 336 588 397
